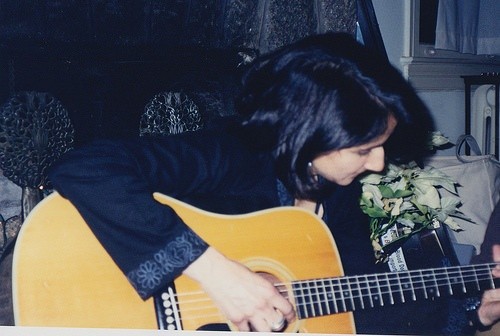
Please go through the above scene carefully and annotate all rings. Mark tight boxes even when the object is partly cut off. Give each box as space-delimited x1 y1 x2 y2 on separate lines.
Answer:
271 315 285 332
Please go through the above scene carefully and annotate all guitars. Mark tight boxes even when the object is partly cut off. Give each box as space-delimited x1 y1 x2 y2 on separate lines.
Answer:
1 187 500 335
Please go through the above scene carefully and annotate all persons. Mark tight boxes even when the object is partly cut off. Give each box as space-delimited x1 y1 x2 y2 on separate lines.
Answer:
42 30 499 336
470 194 499 288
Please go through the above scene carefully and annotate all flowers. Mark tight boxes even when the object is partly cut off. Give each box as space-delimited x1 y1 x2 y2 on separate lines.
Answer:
359 160 472 265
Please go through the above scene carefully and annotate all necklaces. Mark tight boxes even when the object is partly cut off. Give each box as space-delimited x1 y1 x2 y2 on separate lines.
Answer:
310 174 322 215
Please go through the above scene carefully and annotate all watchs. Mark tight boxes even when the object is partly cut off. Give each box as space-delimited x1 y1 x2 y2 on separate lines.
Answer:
463 291 490 332
